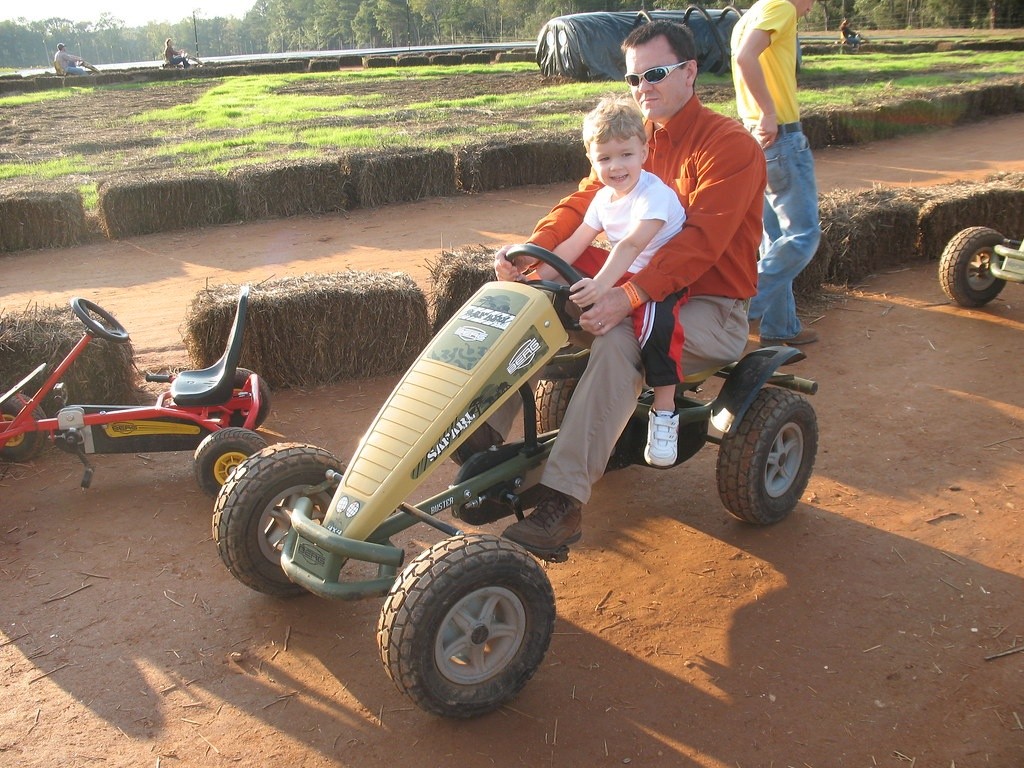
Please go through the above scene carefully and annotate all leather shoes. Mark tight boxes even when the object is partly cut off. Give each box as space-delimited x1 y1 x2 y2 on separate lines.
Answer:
759 328 818 347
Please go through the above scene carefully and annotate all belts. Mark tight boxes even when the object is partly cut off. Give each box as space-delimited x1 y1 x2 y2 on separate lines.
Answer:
746 122 803 134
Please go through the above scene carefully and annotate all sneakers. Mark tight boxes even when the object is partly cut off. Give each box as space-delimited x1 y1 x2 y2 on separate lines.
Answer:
644 403 680 469
448 421 505 467
499 488 583 555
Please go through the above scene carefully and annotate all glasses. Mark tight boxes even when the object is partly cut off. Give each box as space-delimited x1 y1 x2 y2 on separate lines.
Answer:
624 59 690 87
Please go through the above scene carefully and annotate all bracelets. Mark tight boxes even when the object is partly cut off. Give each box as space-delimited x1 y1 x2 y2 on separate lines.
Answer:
621 282 641 311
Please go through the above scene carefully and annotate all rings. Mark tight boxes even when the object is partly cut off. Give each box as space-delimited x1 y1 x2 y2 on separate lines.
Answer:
598 322 603 327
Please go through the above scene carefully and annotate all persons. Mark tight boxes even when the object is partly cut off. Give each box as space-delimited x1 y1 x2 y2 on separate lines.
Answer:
526 97 685 469
841 20 861 47
164 38 190 68
55 43 89 75
730 0 820 345
450 20 766 553
